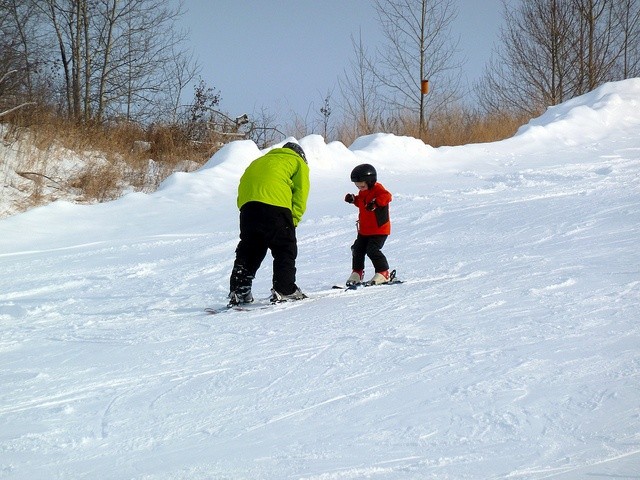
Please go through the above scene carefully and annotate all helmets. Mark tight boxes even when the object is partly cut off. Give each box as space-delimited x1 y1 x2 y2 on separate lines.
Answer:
282 142 308 164
351 164 377 189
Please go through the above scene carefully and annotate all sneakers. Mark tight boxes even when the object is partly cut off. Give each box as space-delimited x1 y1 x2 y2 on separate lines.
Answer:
348 270 364 284
369 269 390 283
229 292 254 304
273 285 308 301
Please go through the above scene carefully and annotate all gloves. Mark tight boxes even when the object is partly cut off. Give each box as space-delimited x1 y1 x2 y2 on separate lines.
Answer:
366 198 376 211
345 193 354 203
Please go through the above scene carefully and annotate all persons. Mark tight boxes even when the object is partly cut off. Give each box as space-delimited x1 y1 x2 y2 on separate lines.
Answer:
227 141 311 307
343 162 393 285
